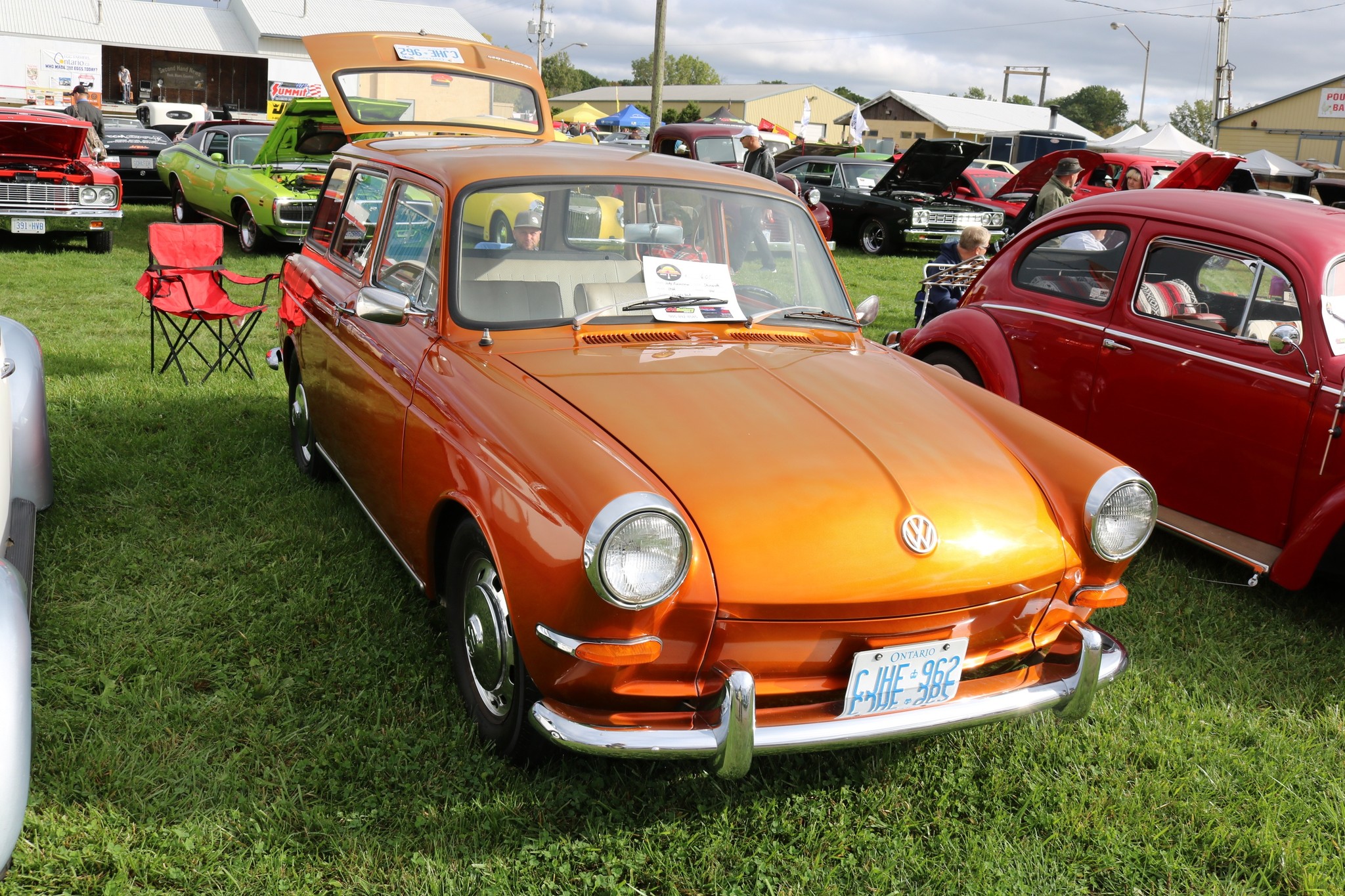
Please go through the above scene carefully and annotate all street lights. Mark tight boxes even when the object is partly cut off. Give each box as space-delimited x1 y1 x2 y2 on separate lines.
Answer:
540 35 587 76
1110 21 1149 129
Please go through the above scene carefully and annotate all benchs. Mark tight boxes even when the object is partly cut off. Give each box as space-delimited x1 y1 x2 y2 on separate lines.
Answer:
1033 274 1125 308
388 257 642 319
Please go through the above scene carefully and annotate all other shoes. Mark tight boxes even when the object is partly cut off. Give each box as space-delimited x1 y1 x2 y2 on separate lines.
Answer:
730 268 738 275
126 102 131 104
760 266 777 273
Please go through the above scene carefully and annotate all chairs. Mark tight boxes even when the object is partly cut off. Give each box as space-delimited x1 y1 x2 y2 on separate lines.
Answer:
574 282 653 316
457 281 562 322
1135 279 1198 316
135 222 281 387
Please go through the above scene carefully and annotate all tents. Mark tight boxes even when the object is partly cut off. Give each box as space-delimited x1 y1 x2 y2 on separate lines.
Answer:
552 102 609 123
596 103 666 133
689 105 759 128
1233 147 1314 189
1085 120 1241 164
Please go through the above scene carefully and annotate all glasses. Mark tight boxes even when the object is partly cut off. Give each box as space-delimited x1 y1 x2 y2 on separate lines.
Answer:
982 244 990 250
664 213 684 221
741 136 747 140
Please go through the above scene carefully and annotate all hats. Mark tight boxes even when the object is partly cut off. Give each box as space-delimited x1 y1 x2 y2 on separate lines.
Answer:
585 124 591 127
736 126 759 138
570 123 575 127
120 66 124 70
633 127 638 130
514 211 542 229
69 85 88 96
1053 157 1086 176
657 201 692 237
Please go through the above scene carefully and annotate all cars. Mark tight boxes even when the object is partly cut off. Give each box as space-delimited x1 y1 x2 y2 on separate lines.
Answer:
417 117 1345 257
156 99 440 258
883 188 1345 616
279 32 1162 783
0 103 265 255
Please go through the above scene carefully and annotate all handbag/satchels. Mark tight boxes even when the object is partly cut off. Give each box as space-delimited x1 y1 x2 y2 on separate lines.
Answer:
1011 191 1039 236
73 104 108 162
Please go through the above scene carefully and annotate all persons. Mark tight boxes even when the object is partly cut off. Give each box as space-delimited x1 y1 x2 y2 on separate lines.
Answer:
927 225 991 314
64 85 105 154
622 126 642 147
645 200 709 263
729 124 777 273
895 143 901 155
843 141 850 145
201 102 214 121
566 121 600 142
1033 157 1154 253
818 135 827 143
221 104 232 121
118 64 132 104
794 136 803 145
502 210 542 252
